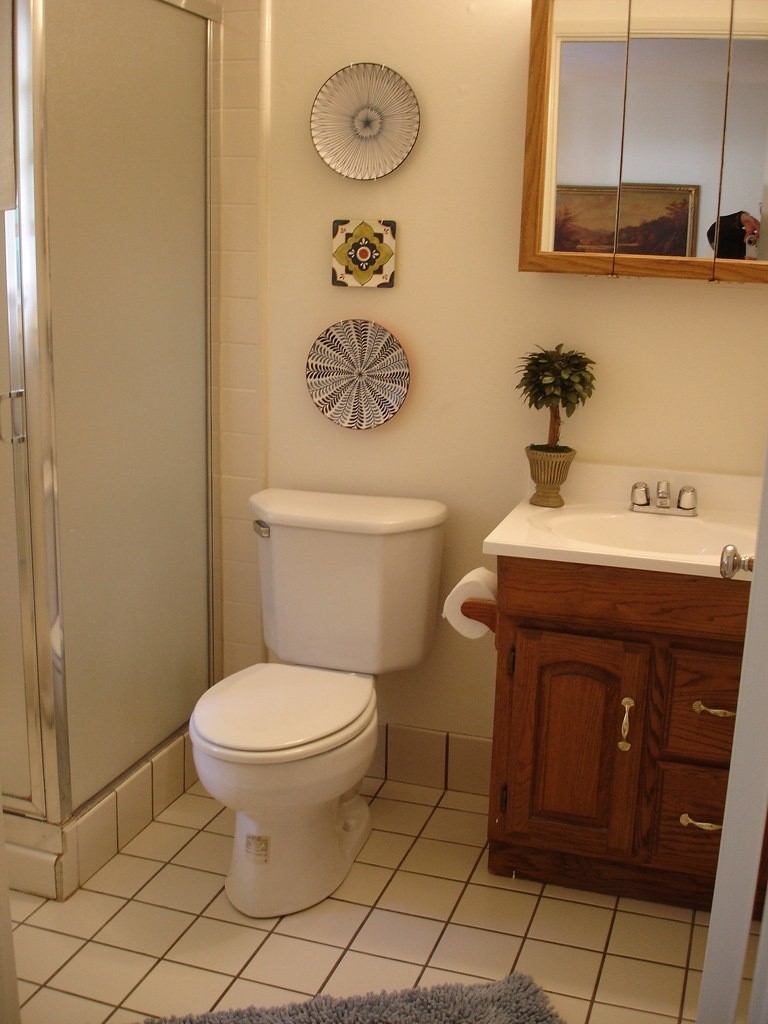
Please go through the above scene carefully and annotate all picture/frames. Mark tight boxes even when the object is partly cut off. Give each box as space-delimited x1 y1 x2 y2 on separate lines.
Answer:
555 182 700 257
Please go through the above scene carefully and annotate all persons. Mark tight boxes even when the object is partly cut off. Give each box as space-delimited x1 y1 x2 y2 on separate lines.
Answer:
706 203 762 260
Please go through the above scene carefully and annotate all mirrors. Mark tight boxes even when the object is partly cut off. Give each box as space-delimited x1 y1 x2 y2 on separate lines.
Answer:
518 0 768 285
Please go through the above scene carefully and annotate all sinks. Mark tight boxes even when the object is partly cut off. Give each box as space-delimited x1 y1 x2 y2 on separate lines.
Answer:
526 511 758 557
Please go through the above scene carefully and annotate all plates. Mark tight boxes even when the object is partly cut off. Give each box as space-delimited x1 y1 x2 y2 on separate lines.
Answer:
330 217 397 289
310 63 420 181
307 319 410 431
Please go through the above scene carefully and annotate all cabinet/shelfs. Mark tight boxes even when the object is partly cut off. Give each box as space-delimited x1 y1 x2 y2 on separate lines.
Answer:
459 554 768 922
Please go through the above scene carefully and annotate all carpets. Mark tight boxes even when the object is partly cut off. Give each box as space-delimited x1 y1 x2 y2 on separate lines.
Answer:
141 970 567 1024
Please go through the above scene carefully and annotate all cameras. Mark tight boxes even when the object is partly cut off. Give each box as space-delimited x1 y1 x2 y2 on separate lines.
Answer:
745 235 758 259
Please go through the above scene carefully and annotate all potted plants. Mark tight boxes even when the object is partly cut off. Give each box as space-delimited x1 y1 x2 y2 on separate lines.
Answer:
513 342 597 510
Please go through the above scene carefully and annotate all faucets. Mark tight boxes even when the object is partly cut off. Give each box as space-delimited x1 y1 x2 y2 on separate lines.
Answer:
656 480 671 508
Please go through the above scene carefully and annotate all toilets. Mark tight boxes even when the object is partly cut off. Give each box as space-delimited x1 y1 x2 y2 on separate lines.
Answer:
187 487 456 921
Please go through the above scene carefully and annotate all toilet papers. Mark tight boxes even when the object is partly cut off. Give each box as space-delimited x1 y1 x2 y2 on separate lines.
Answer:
441 567 497 640
